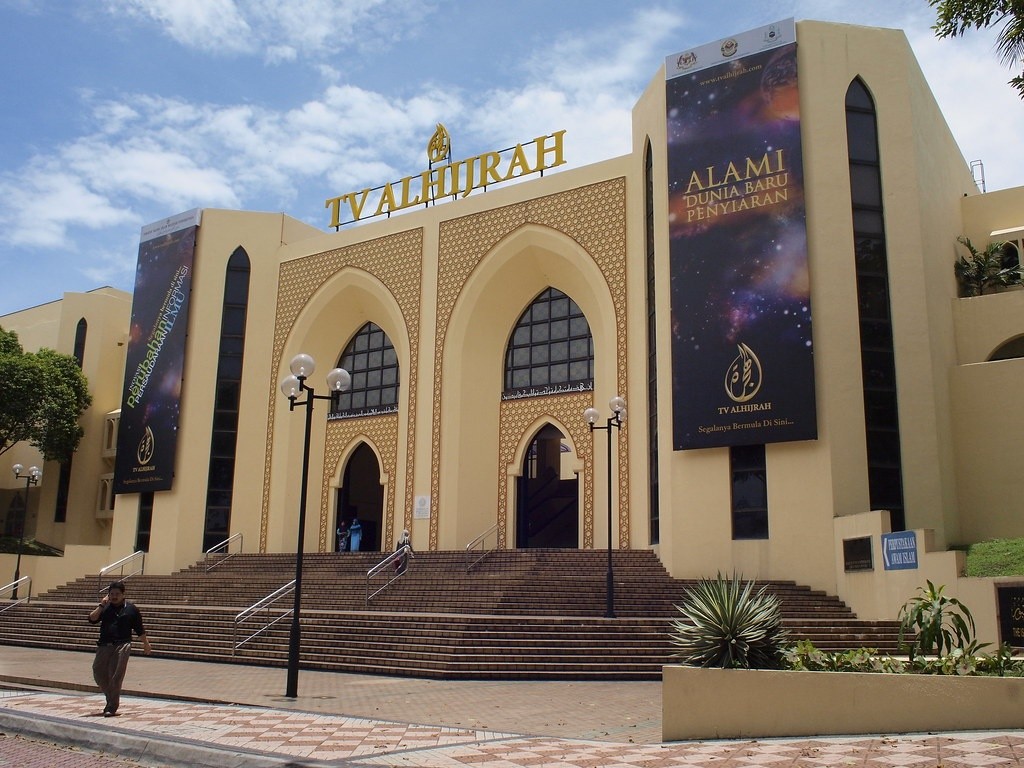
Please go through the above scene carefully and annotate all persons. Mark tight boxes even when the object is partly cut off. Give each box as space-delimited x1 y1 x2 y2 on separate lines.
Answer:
337 521 349 552
396 529 415 575
87 582 150 715
349 519 362 552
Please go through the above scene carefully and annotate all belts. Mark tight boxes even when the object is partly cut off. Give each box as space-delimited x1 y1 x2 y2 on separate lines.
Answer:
99 640 129 647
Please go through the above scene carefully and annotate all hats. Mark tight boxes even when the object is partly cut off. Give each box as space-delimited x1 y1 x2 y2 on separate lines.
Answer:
352 519 358 522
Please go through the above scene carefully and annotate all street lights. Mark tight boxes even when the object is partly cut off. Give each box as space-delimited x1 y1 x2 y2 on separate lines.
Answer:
280 354 352 698
10 464 43 600
584 397 628 618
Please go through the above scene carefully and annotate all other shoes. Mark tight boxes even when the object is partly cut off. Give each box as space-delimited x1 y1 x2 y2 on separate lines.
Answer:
103 707 116 716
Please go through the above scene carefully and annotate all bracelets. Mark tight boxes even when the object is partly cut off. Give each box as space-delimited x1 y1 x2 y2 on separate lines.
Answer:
98 604 104 608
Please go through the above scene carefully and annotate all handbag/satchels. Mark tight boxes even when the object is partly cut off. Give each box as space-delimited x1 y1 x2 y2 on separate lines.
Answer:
404 537 411 555
392 561 400 570
